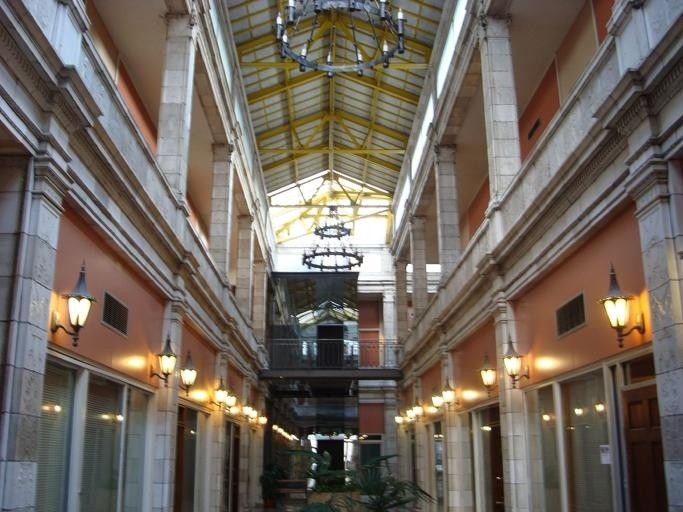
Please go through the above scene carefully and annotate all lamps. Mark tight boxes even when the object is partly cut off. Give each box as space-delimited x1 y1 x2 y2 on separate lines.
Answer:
595 265 648 352
47 261 96 350
148 326 200 400
479 332 533 396
210 375 257 423
405 376 459 421
